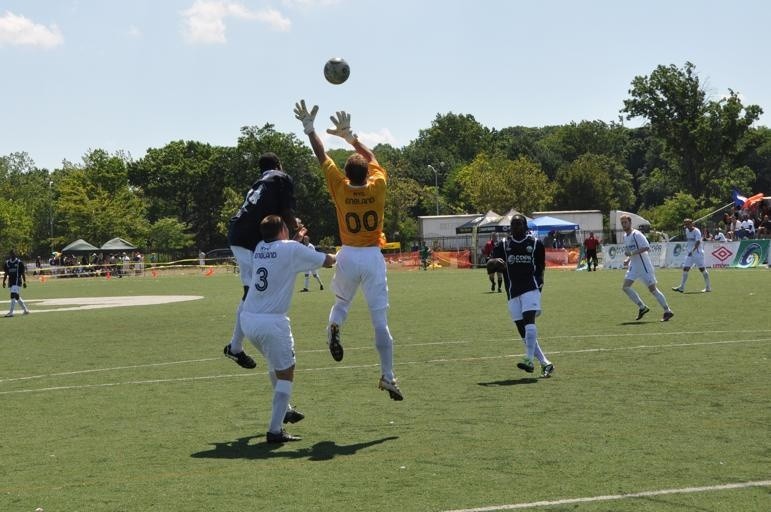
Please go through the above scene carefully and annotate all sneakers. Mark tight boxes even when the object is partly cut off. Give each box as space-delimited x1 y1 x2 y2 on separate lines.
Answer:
636 305 649 320
517 362 534 372
224 344 256 368
540 363 554 378
672 287 683 292
702 288 711 291
379 378 403 401
283 410 305 424
660 312 673 322
327 323 343 361
267 428 302 443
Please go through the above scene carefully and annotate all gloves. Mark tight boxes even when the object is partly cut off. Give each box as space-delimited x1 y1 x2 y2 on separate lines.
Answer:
325 111 357 146
293 99 318 136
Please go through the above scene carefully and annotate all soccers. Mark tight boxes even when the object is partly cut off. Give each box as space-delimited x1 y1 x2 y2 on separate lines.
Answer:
324 58 349 84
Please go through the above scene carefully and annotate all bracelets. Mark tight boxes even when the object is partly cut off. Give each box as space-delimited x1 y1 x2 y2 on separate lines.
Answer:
23 281 27 284
630 251 635 257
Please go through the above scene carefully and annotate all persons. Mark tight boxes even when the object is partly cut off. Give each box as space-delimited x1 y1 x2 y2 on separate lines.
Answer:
619 214 674 322
672 218 712 293
544 231 603 272
703 200 771 241
239 214 336 445
294 99 403 402
33 251 158 278
487 214 555 378
482 232 502 293
199 252 206 272
3 249 30 317
411 241 441 270
224 151 303 369
301 236 324 292
